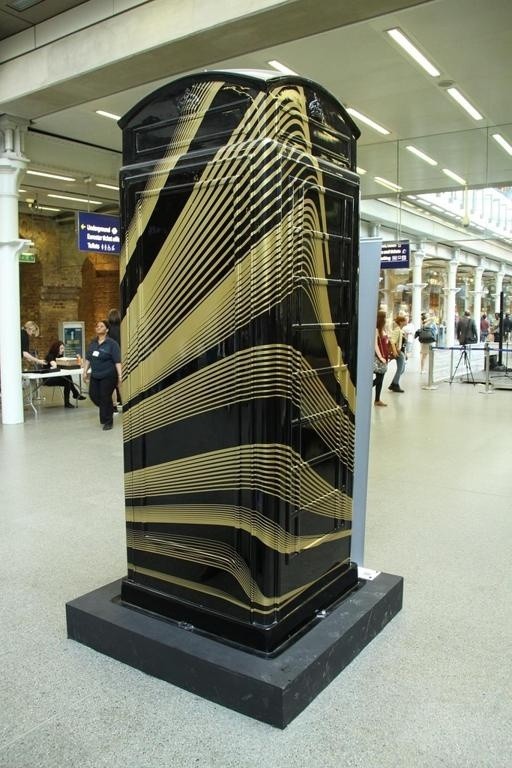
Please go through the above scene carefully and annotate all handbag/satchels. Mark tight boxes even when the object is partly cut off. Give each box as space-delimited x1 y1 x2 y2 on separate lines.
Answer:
388 340 400 359
465 337 478 344
373 354 387 373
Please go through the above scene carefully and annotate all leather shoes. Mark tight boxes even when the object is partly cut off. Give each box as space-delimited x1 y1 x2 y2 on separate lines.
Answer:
103 424 112 430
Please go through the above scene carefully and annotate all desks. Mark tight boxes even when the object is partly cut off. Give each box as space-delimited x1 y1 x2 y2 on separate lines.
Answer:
22 366 92 422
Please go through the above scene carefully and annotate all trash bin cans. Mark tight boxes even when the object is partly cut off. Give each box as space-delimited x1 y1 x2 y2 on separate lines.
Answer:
485 355 497 372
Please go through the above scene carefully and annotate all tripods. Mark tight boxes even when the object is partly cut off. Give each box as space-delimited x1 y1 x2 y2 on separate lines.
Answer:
491 342 512 381
449 350 474 385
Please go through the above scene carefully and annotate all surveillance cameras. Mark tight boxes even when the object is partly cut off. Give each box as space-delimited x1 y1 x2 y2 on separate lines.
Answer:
462 217 470 227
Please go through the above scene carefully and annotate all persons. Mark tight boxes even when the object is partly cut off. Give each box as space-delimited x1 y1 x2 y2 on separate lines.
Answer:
373 309 512 407
22 321 47 366
108 308 122 405
83 319 121 430
43 342 86 408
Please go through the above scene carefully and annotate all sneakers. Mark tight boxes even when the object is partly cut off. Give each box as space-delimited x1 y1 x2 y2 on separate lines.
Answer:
374 400 387 406
388 383 404 392
73 393 86 400
65 402 75 408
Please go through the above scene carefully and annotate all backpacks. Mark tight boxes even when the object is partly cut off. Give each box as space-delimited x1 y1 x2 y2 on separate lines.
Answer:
418 324 435 343
